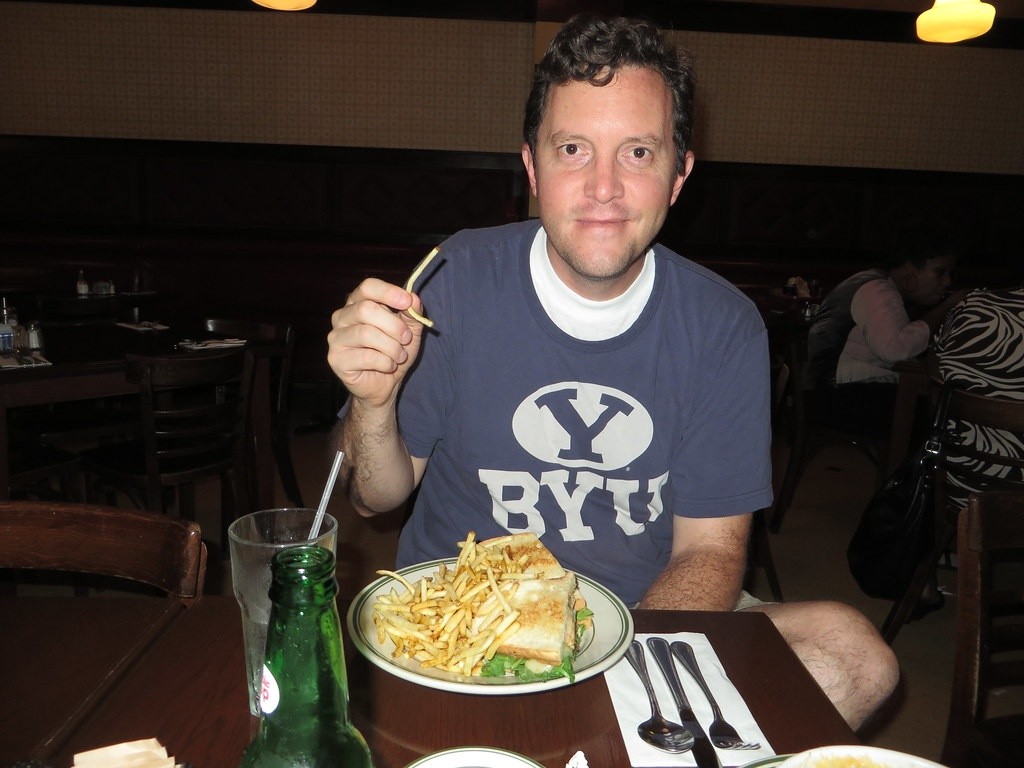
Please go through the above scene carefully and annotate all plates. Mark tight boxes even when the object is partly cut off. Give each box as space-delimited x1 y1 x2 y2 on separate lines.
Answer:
346 557 634 695
402 745 545 768
736 752 796 768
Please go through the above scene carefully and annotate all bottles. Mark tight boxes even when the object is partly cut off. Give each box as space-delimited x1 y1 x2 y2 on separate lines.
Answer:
239 544 374 767
804 304 812 316
28 320 44 350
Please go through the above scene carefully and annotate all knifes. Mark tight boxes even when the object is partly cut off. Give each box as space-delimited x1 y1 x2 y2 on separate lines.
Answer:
647 638 723 767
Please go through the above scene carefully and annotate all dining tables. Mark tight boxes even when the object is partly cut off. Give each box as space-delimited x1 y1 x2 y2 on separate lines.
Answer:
2 317 250 410
40 599 860 768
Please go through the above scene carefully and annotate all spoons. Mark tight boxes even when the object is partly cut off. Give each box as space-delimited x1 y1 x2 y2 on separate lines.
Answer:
624 638 695 754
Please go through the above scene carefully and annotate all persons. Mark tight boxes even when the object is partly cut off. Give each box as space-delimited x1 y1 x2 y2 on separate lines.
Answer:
326 9 901 731
801 229 1024 623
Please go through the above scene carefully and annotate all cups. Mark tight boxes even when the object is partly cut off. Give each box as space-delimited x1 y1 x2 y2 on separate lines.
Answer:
228 507 338 718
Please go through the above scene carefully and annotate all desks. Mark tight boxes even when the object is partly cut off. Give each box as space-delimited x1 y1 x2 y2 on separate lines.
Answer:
1 591 185 768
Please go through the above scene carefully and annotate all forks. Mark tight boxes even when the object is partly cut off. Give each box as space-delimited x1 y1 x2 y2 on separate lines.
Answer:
670 641 761 750
17 344 47 364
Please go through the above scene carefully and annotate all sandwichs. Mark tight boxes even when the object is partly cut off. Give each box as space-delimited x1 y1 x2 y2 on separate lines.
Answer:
475 531 596 685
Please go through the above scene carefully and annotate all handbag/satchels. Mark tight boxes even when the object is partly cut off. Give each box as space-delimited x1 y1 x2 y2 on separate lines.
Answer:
845 380 952 604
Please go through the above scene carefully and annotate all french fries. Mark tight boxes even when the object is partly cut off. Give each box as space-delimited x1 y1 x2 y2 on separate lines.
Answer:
407 246 441 327
371 531 540 677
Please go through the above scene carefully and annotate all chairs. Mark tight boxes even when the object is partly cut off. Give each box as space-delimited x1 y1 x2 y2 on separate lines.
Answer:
0 257 1024 768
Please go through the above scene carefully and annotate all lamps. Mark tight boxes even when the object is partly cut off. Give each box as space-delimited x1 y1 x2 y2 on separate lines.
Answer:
917 0 996 44
